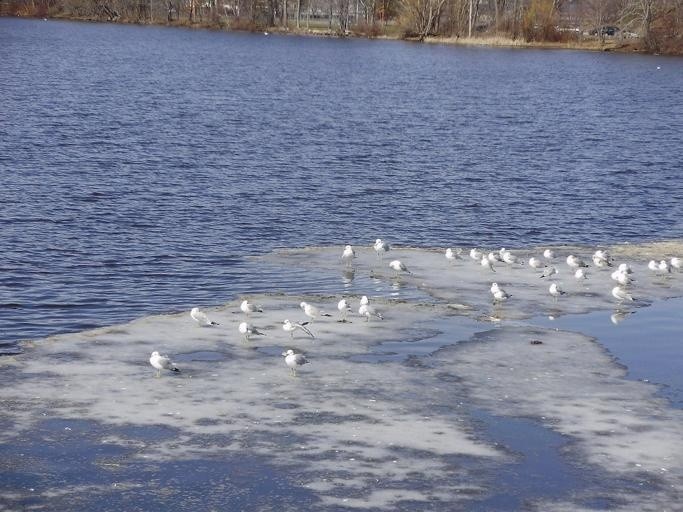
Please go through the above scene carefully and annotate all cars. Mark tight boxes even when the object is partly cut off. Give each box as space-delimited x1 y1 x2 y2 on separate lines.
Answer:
587 24 622 38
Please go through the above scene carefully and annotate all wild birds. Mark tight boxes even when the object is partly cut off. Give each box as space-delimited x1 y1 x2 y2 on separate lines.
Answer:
148 350 181 378
469 246 682 302
189 306 219 327
340 245 358 263
387 259 412 276
445 247 461 266
373 238 390 257
285 349 311 376
610 308 637 325
238 295 379 341
